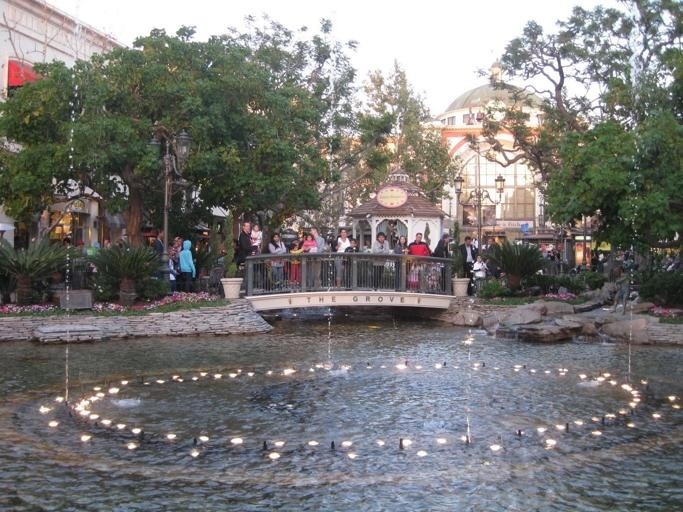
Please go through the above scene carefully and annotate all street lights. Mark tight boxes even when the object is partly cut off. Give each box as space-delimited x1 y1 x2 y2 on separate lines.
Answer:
146 120 192 293
450 174 506 299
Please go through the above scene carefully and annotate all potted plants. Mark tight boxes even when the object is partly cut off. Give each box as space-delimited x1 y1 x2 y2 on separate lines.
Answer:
219 218 244 298
450 219 472 296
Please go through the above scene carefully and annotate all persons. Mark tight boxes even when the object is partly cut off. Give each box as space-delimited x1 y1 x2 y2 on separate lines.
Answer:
574 246 628 274
64 235 111 273
543 245 564 272
